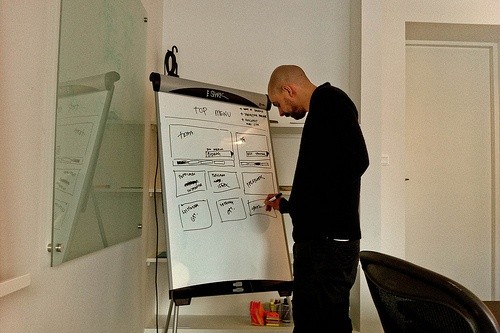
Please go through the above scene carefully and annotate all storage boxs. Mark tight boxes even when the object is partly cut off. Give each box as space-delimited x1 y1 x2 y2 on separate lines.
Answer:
249 299 293 326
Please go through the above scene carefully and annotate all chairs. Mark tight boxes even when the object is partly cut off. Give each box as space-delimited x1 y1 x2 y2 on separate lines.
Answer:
360 251 500 332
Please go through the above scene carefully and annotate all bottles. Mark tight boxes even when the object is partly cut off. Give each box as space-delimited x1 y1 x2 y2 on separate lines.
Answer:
270 299 274 311
274 300 279 313
281 299 290 323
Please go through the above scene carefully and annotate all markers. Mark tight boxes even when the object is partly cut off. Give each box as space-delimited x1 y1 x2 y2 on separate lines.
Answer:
261 193 282 207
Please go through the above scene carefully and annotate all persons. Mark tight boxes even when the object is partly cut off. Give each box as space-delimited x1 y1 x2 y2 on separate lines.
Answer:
263 64 370 333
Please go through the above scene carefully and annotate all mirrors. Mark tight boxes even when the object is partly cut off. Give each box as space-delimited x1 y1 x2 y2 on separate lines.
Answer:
51 0 147 266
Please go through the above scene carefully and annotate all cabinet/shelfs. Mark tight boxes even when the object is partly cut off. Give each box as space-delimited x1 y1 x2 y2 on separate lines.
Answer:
145 120 304 332
